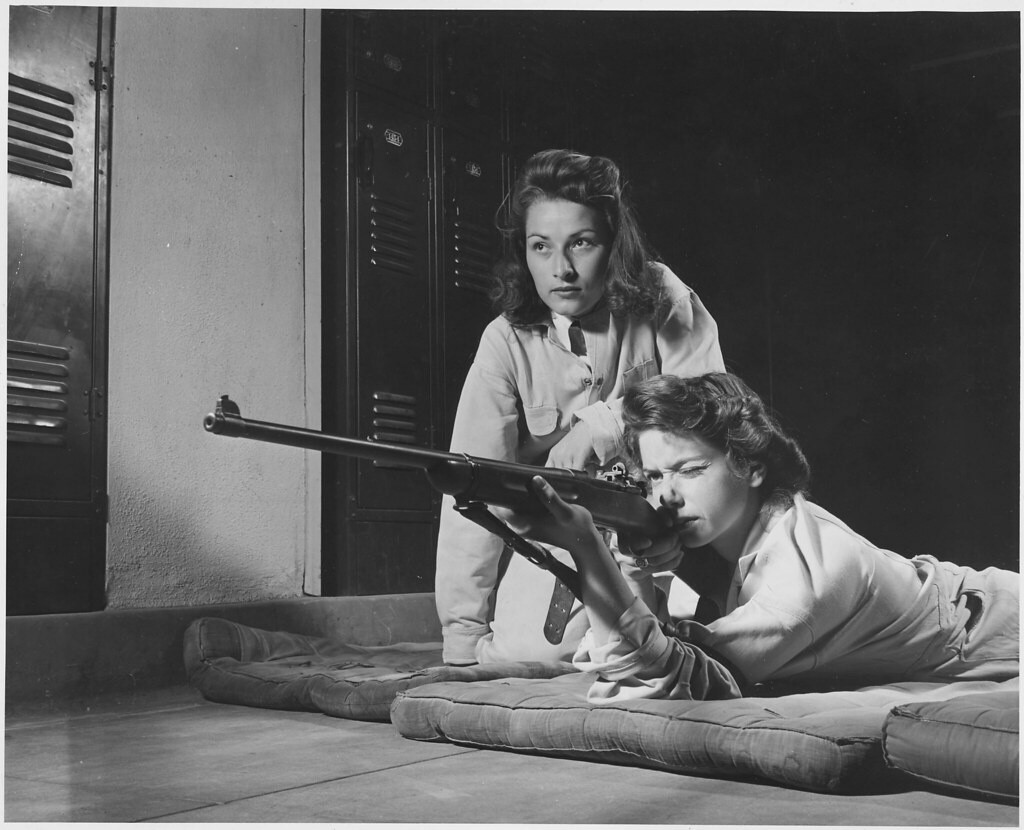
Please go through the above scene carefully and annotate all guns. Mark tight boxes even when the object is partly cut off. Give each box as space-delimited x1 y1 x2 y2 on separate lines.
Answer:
201 391 732 610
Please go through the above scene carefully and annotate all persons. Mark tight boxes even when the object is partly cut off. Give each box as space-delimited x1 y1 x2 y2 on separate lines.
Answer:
434 151 728 666
502 368 1019 704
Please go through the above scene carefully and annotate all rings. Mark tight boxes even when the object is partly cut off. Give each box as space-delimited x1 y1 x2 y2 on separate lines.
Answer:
633 557 649 568
